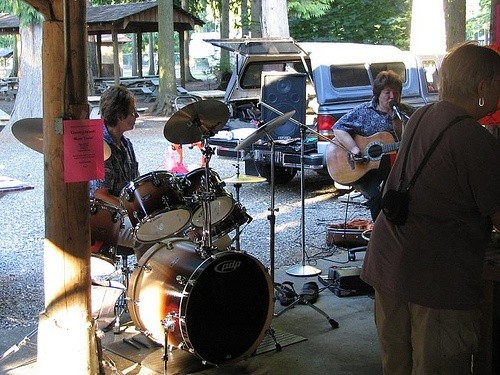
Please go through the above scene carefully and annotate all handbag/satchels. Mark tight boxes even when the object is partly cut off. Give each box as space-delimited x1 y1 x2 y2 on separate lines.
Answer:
381 189 409 226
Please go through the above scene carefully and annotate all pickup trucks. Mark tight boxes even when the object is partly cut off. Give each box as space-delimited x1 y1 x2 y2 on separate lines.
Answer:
198 37 448 185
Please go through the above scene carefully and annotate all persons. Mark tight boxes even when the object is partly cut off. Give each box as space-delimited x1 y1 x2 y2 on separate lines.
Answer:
88 85 156 261
332 71 415 222
361 43 500 375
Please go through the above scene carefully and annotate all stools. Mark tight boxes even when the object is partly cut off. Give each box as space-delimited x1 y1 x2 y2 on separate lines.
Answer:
115 245 138 313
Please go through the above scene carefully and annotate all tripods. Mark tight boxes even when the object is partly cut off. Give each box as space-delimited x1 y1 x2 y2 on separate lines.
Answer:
268 141 338 329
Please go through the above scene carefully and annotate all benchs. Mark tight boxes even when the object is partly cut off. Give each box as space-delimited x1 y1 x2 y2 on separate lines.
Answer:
0 109 10 125
125 88 187 95
88 107 147 119
172 103 187 110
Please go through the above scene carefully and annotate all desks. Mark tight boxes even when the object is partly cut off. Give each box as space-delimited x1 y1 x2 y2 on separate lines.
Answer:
93 76 159 87
188 89 227 101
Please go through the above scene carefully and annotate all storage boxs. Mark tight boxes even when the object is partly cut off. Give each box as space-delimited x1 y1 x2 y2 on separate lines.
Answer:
326 225 373 246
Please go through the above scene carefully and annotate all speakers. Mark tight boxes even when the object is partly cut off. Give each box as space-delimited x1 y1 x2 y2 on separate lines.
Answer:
261 71 308 140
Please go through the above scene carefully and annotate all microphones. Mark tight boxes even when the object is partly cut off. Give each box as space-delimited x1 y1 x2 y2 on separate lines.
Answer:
355 156 370 164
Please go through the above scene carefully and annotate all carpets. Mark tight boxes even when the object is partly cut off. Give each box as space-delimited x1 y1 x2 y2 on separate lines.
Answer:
103 318 306 375
